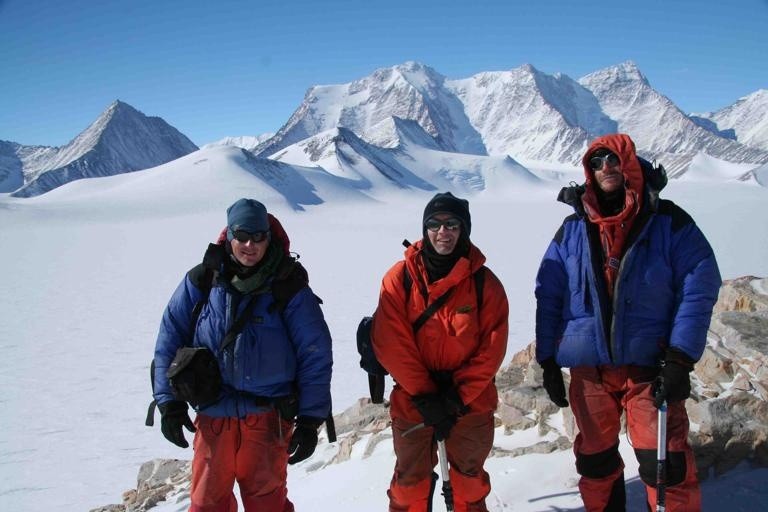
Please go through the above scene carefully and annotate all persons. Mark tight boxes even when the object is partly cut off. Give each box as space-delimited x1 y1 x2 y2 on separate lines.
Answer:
370 190 511 512
150 197 333 512
534 133 723 511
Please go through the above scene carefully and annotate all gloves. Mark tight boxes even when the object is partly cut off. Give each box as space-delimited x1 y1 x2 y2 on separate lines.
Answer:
287 415 323 465
411 380 473 442
648 345 696 410
157 399 198 449
540 361 570 407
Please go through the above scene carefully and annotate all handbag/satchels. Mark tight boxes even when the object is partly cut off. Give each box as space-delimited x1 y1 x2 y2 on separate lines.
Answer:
165 344 227 409
357 316 389 378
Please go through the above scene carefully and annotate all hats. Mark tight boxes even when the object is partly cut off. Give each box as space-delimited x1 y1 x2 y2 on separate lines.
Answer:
422 191 471 250
226 198 270 242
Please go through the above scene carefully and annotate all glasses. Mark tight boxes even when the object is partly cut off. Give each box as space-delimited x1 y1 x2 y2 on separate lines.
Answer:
426 216 463 232
588 154 621 171
229 226 269 243
